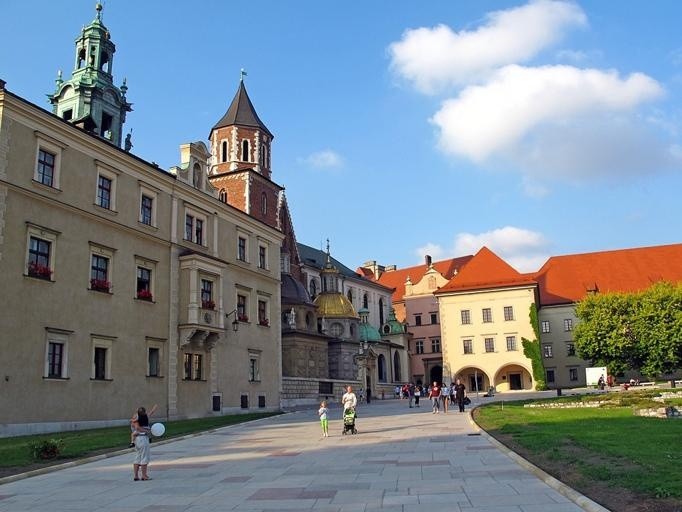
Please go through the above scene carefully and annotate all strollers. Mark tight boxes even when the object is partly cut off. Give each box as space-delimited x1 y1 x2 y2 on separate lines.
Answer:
342 405 357 435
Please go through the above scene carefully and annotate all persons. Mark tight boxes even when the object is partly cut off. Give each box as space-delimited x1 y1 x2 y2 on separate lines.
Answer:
124 133 135 152
597 374 607 390
366 386 374 406
630 378 636 386
129 403 159 482
392 379 471 415
634 378 641 387
358 387 365 405
318 401 335 437
607 375 615 388
342 386 357 434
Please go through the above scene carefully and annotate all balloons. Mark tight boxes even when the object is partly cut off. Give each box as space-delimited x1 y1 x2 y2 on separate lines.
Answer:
148 422 168 439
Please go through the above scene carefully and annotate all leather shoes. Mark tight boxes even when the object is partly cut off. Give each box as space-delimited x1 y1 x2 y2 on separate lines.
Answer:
142 478 151 481
134 478 139 481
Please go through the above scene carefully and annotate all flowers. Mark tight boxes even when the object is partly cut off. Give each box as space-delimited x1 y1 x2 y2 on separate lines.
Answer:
89 279 109 288
238 313 248 321
136 289 152 299
201 299 214 308
27 260 51 274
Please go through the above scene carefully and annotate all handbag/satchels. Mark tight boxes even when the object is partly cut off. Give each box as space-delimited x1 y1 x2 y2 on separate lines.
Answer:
463 396 471 405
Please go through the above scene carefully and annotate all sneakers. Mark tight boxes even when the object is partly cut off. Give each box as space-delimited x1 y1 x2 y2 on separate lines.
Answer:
128 443 135 448
324 433 328 437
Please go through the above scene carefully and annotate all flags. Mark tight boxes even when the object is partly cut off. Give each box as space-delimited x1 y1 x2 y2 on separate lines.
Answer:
242 68 248 77
